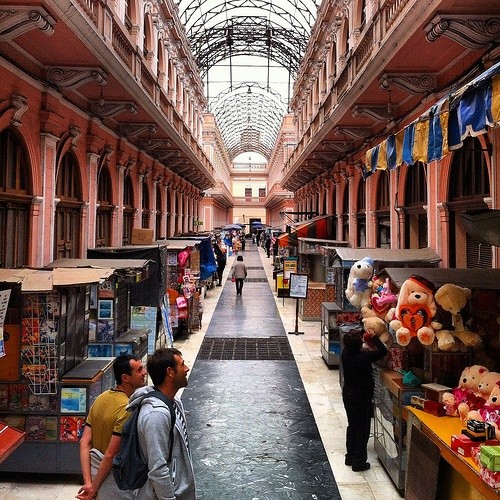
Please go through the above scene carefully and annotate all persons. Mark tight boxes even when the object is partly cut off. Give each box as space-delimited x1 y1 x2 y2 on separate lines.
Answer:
126 348 196 500
214 231 272 296
338 329 387 472
74 355 148 500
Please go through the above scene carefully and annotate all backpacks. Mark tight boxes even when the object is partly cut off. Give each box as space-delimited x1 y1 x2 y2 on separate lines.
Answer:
111 390 176 491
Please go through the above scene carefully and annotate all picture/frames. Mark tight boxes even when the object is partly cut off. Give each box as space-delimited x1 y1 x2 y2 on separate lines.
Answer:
97 298 113 320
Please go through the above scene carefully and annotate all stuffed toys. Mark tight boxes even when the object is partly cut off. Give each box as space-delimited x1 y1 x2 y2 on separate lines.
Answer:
344 257 500 440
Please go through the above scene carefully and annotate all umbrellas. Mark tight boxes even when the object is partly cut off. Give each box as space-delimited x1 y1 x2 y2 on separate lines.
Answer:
222 222 266 230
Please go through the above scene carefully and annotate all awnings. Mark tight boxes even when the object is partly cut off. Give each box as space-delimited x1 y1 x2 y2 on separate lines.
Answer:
278 211 333 247
363 62 500 179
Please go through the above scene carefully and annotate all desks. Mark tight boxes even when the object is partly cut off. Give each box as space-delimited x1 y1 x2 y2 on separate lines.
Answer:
401 405 500 500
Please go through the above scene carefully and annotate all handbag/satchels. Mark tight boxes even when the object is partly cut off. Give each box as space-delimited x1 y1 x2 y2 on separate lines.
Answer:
231 277 235 282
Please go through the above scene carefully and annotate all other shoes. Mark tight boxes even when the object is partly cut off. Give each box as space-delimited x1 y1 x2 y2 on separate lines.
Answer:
345 456 370 471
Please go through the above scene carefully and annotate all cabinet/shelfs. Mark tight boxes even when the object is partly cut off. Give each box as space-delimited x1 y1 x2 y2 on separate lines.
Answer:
320 301 343 366
115 328 148 386
374 367 424 489
0 356 116 486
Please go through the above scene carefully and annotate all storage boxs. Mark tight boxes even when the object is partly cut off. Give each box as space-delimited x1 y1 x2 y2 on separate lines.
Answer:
131 227 154 245
411 383 500 489
387 349 408 372
